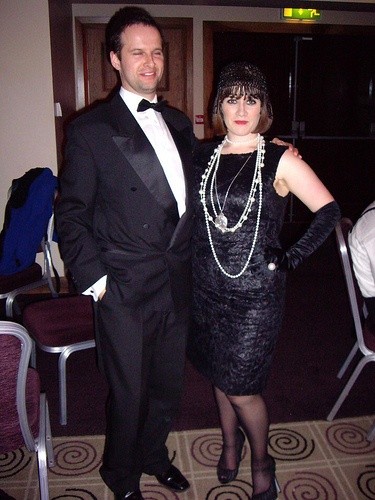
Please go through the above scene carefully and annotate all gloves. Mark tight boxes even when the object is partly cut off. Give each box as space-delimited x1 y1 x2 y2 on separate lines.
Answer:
266 201 341 273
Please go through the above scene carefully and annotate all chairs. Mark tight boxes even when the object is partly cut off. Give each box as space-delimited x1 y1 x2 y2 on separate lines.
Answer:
0 168 96 500
326 217 375 421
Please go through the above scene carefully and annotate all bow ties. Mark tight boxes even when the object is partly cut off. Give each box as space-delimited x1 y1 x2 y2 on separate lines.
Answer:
136 99 169 113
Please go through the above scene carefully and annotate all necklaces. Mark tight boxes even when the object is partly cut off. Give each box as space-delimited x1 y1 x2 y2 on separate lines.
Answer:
199 133 266 277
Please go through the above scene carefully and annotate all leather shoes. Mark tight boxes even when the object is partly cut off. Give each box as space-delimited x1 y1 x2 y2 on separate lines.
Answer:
100 466 143 500
145 462 189 492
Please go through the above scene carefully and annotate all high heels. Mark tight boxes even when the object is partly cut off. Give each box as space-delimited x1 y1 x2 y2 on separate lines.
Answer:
217 429 245 484
251 455 281 500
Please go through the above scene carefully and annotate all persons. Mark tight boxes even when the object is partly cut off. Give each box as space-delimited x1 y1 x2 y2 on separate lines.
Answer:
186 59 339 500
54 7 302 500
348 200 375 337
